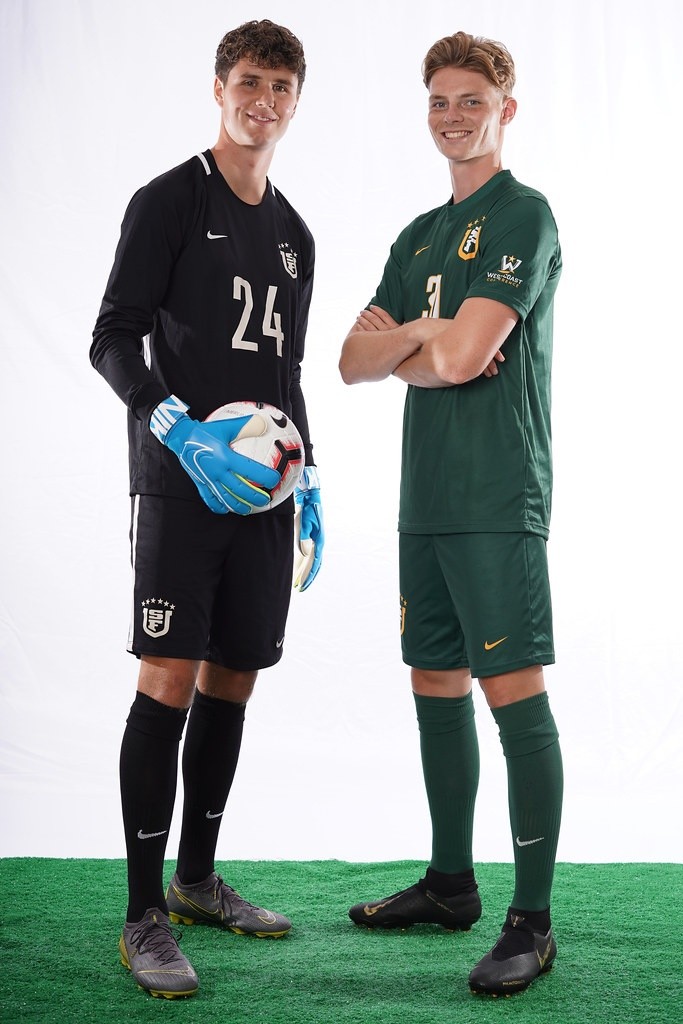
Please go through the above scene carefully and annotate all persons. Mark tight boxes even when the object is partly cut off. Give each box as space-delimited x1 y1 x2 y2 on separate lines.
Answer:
84 18 328 1000
342 30 567 997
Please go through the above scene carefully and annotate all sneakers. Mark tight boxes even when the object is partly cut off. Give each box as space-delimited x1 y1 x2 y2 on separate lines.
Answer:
116 871 291 999
348 867 558 997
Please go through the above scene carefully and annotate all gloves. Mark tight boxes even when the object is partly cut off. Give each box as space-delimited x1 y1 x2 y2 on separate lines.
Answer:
148 394 282 514
292 466 325 592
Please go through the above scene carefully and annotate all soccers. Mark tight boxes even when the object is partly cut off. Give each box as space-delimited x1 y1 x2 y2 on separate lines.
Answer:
201 400 306 514
